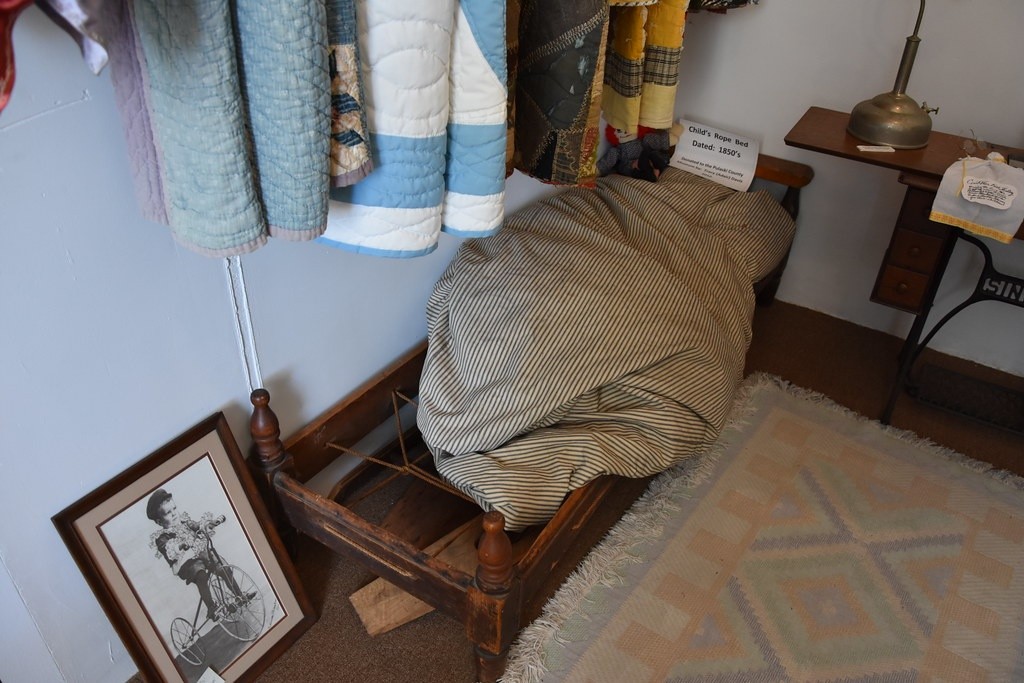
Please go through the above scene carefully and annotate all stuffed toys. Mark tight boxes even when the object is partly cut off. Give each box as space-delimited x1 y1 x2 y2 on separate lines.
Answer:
595 124 684 182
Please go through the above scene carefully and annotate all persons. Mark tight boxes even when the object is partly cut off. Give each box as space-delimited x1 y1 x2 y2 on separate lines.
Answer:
146 489 258 622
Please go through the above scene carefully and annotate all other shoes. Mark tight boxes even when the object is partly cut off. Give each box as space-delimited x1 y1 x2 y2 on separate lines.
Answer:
235 592 256 607
208 605 221 621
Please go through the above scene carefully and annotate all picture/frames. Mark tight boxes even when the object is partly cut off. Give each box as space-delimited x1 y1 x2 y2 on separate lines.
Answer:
50 410 319 683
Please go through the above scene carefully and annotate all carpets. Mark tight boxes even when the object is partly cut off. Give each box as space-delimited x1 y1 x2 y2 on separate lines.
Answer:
495 370 1024 683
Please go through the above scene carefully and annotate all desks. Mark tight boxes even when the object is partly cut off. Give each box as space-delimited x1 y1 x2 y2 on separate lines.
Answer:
784 106 1024 424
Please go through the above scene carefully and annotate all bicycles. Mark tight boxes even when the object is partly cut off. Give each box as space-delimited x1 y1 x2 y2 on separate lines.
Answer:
171 513 266 666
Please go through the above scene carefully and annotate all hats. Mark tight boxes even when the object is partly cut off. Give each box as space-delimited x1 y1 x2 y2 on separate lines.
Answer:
147 488 172 519
